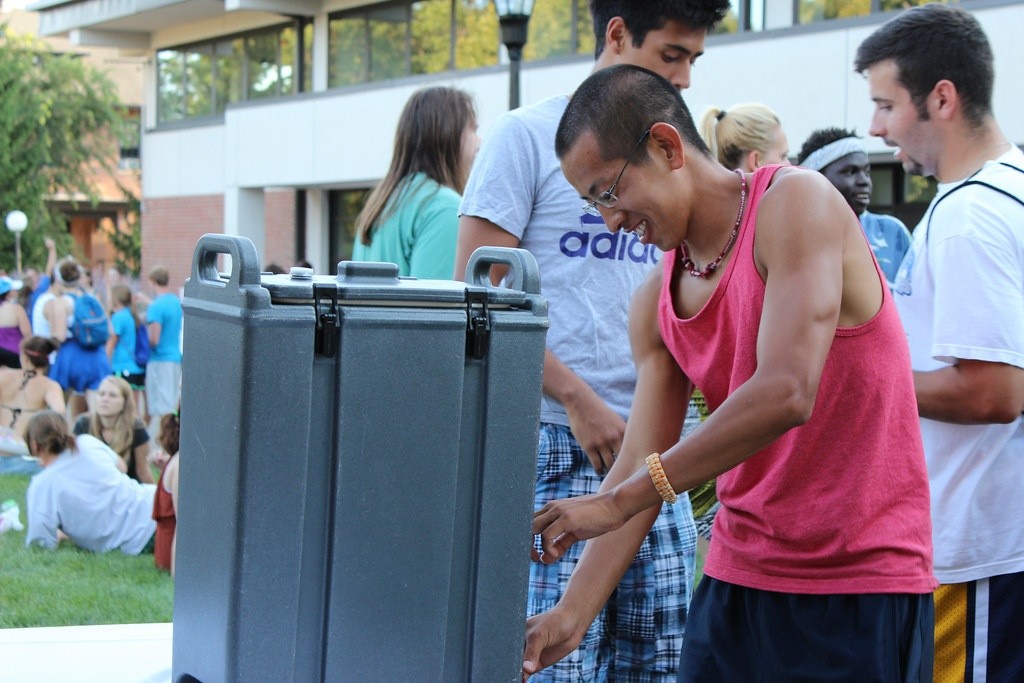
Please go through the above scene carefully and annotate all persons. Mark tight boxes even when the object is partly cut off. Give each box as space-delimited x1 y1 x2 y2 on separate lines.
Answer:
856 4 1024 683
351 86 482 286
453 0 731 683
520 64 934 683
0 258 314 579
797 128 914 291
703 105 793 174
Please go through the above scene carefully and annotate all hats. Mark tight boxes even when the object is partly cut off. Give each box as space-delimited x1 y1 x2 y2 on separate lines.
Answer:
0 276 24 296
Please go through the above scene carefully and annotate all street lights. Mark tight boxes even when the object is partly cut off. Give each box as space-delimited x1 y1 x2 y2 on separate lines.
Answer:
488 0 540 112
5 210 29 275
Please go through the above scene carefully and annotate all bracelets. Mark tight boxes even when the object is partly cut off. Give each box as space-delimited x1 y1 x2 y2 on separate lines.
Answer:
645 452 676 503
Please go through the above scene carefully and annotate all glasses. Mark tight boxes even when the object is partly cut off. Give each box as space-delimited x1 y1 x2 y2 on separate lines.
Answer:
583 130 650 217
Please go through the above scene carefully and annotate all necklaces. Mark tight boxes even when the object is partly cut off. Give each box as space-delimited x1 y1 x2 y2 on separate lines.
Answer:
680 169 746 279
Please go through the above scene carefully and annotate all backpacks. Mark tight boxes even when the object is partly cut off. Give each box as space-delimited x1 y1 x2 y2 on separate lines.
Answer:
121 314 152 367
64 285 110 347
